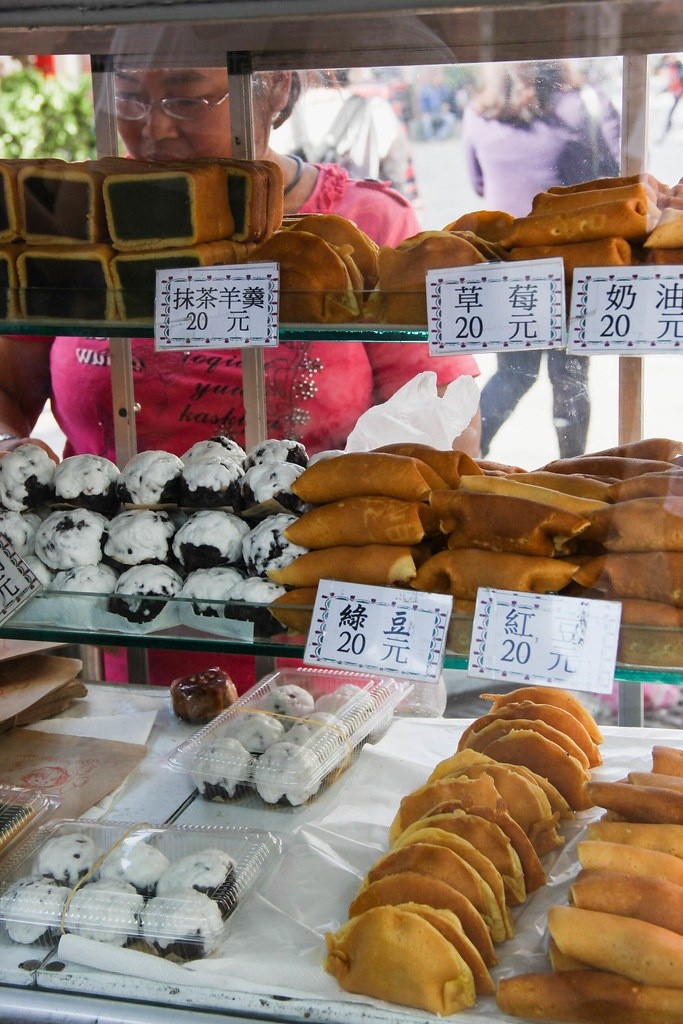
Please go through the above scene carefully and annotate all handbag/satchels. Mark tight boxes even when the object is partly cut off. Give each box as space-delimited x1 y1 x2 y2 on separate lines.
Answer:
559 89 619 187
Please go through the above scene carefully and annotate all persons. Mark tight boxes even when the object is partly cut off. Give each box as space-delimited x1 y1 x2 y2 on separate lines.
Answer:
451 54 683 459
0 69 481 718
420 67 477 138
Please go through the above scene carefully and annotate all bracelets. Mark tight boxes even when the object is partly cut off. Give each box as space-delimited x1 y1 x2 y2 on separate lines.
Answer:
0 433 16 441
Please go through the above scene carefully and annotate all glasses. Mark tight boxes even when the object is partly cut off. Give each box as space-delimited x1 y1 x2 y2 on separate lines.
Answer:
115 92 229 121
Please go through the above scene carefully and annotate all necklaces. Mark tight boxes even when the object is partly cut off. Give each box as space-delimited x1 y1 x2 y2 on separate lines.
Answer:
284 154 302 195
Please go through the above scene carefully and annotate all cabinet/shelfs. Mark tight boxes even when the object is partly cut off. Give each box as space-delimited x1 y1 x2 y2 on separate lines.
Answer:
0 0 683 686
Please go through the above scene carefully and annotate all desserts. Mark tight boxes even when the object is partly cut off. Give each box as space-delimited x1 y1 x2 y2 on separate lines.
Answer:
170 668 379 806
318 685 683 1024
245 174 682 326
0 153 286 325
0 832 242 966
0 431 355 641
265 436 683 668
